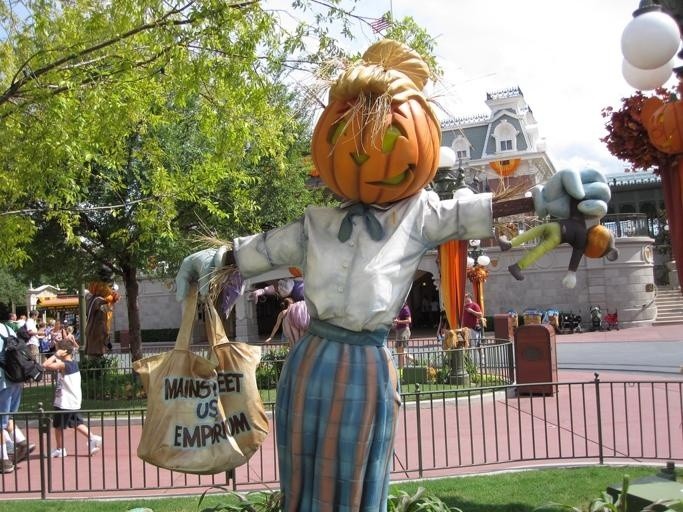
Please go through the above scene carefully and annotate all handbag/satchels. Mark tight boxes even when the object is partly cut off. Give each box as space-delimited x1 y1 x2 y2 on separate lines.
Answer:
132 286 272 476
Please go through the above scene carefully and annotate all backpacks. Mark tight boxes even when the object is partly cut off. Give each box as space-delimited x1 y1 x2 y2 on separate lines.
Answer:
1 324 42 383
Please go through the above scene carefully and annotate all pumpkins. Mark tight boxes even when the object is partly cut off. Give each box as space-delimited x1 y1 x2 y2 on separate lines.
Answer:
584 224 619 261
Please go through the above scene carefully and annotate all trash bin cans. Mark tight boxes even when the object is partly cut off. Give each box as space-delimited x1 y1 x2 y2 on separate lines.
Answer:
120 331 130 352
493 313 512 345
513 323 559 397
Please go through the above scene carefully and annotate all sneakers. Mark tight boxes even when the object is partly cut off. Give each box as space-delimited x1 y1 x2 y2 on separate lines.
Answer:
47 445 68 461
12 438 35 466
1 457 15 476
87 433 106 458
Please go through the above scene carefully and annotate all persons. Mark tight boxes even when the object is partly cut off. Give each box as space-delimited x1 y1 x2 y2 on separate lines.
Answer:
500 212 622 288
434 302 450 339
0 308 102 476
263 295 299 349
242 277 309 349
83 281 113 359
172 32 609 511
391 300 418 371
462 291 483 330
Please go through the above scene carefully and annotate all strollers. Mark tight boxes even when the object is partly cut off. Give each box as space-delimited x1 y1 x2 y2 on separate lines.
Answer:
589 304 619 332
506 309 583 334
